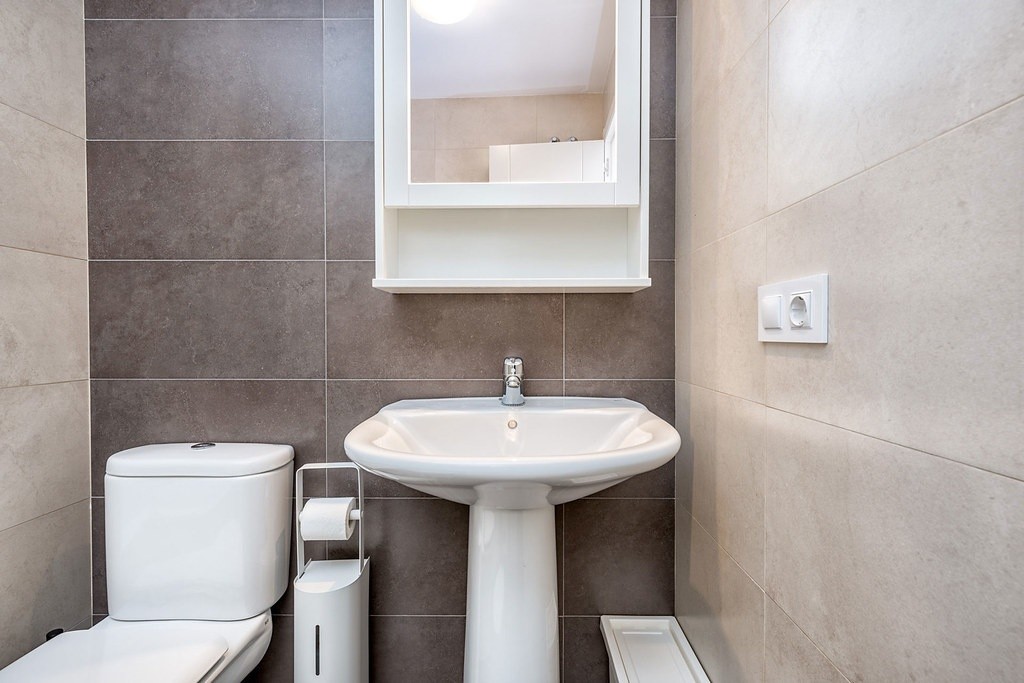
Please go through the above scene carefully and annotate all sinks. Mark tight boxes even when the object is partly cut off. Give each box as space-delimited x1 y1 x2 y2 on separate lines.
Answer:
343 394 682 510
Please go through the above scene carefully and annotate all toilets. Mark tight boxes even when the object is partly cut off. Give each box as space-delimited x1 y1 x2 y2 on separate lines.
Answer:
0 438 295 683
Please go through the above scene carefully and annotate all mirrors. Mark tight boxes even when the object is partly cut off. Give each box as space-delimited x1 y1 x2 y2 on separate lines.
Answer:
408 0 618 185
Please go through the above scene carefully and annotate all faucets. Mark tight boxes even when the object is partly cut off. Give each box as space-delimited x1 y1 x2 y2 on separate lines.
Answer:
500 355 526 406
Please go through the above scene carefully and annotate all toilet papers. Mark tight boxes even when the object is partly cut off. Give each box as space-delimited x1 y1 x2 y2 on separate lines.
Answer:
299 495 357 540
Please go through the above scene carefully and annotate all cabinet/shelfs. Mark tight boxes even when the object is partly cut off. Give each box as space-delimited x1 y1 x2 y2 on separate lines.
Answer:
372 0 651 294
